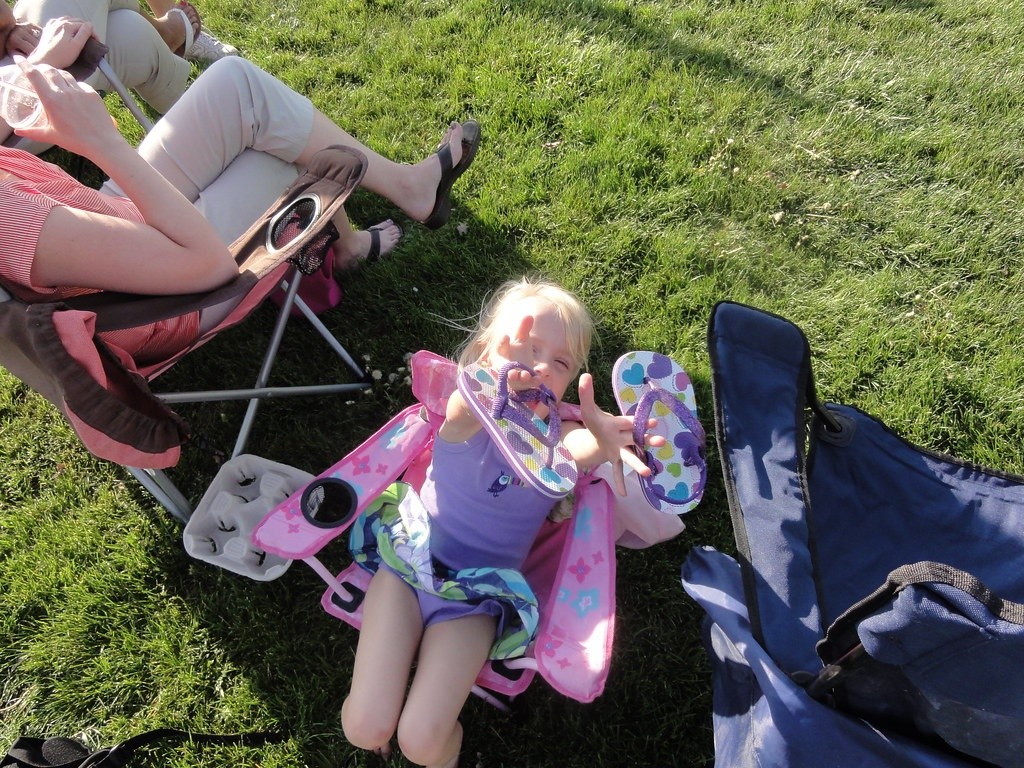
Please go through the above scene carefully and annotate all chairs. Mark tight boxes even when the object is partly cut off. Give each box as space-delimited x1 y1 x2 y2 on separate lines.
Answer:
253 349 616 713
0 37 372 526
681 300 1024 767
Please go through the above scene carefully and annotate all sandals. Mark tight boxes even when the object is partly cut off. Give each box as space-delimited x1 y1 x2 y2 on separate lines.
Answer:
612 351 707 515
456 360 578 500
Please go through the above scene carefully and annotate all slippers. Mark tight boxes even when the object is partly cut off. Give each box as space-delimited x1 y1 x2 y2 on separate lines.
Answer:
420 119 481 231
338 219 403 281
165 3 201 58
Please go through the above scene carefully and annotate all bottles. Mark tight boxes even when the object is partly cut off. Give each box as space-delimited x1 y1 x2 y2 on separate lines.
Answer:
0 64 118 130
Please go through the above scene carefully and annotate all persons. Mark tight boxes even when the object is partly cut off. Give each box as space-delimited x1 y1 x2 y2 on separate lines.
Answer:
341 283 665 768
145 0 238 61
0 55 481 363
0 0 202 116
